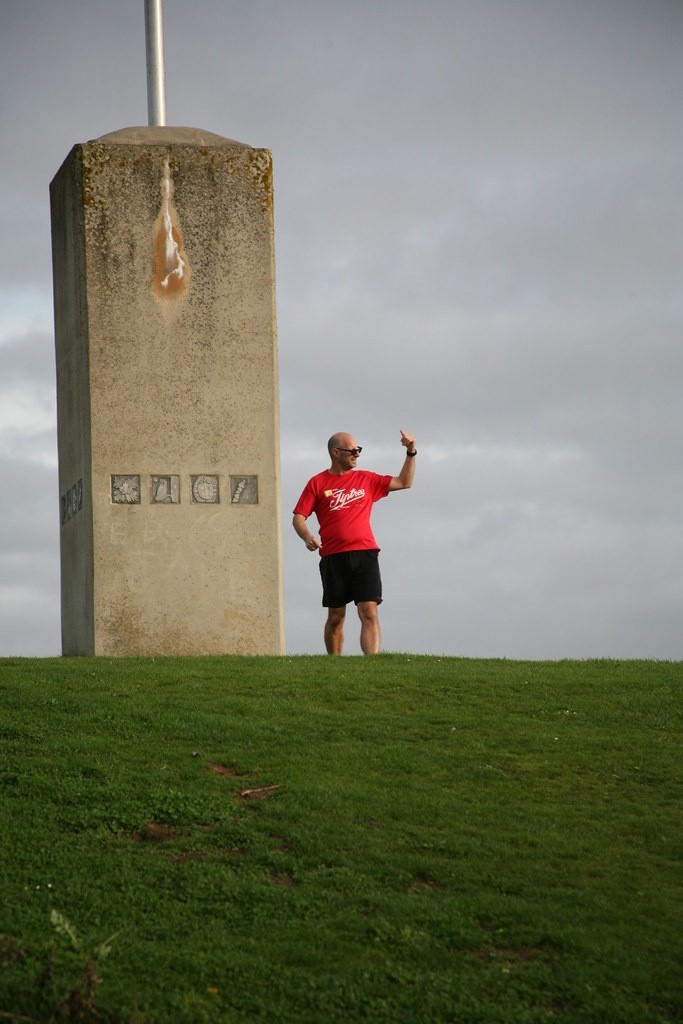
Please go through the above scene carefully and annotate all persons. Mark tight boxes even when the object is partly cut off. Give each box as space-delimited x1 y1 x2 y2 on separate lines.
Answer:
292 429 415 658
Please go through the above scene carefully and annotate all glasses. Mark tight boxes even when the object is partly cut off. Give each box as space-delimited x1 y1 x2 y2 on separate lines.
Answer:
336 446 363 455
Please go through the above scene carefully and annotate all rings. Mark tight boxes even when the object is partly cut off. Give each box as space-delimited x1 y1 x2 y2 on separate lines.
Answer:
312 547 314 549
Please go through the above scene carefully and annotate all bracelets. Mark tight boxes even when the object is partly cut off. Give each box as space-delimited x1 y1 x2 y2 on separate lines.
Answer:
407 449 417 456
